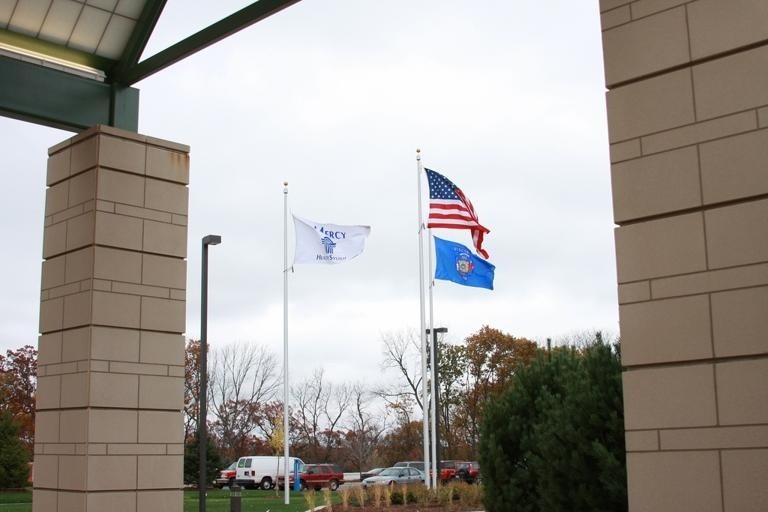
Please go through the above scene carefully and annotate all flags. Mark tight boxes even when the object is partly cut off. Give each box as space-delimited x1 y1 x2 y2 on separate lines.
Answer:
291 213 372 267
431 234 496 291
422 168 491 260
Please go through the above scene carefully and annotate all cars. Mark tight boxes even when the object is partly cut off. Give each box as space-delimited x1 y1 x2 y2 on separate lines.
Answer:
213 456 480 490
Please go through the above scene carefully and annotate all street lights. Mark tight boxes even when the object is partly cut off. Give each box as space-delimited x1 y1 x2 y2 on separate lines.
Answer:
200 235 221 511
426 328 448 480
